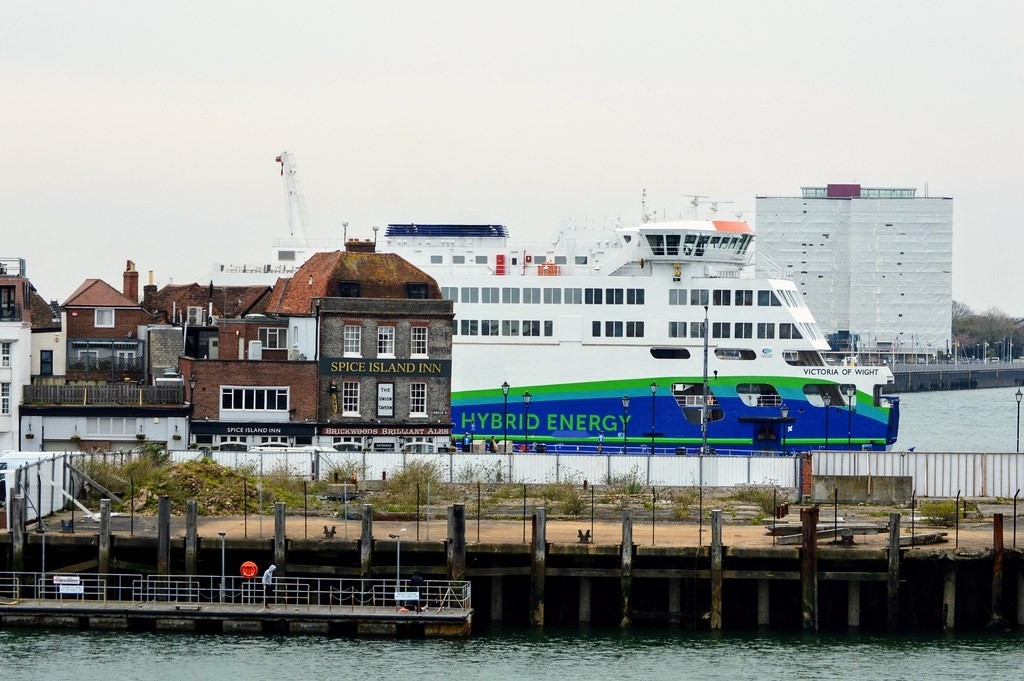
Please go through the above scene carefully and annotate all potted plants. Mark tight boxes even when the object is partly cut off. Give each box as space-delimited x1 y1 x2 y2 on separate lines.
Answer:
72 436 80 442
136 434 145 440
173 435 182 441
26 434 34 439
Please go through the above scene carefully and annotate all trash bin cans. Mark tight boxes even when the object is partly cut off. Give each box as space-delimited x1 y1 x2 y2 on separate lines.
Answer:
841 530 853 546
462 444 470 453
472 439 486 454
537 443 546 453
497 440 512 454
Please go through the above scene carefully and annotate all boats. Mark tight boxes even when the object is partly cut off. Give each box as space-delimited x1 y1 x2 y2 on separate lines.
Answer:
384 189 900 457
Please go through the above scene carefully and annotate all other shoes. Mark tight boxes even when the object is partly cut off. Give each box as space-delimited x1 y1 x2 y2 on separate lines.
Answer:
266 605 271 609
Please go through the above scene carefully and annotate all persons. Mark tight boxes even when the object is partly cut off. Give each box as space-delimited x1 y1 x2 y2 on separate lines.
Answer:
409 570 424 612
452 432 497 453
261 563 277 609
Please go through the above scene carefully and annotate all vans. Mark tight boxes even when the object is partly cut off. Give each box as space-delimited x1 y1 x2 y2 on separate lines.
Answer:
986 357 999 362
0 450 86 521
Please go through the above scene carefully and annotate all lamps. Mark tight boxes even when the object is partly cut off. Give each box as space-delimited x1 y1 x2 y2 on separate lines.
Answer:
367 434 373 448
290 437 294 448
329 384 338 395
399 435 405 449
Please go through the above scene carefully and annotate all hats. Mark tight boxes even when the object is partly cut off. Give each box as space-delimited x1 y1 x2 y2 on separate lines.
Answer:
465 432 468 435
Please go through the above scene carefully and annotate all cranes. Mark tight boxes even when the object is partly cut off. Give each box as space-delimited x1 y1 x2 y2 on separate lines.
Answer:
276 151 312 249
220 249 306 274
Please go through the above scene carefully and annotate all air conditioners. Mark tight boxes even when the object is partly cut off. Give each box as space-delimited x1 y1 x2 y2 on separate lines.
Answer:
187 306 203 326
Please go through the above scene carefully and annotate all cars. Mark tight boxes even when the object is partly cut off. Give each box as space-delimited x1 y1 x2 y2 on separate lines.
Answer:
917 358 926 364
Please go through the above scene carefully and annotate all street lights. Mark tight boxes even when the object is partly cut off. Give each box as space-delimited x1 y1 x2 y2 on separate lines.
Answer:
218 531 225 603
822 393 832 450
501 381 510 455
389 534 400 606
36 529 45 599
845 385 854 451
188 375 197 450
621 395 631 454
780 404 789 457
650 381 658 455
522 391 532 453
1015 387 1023 452
